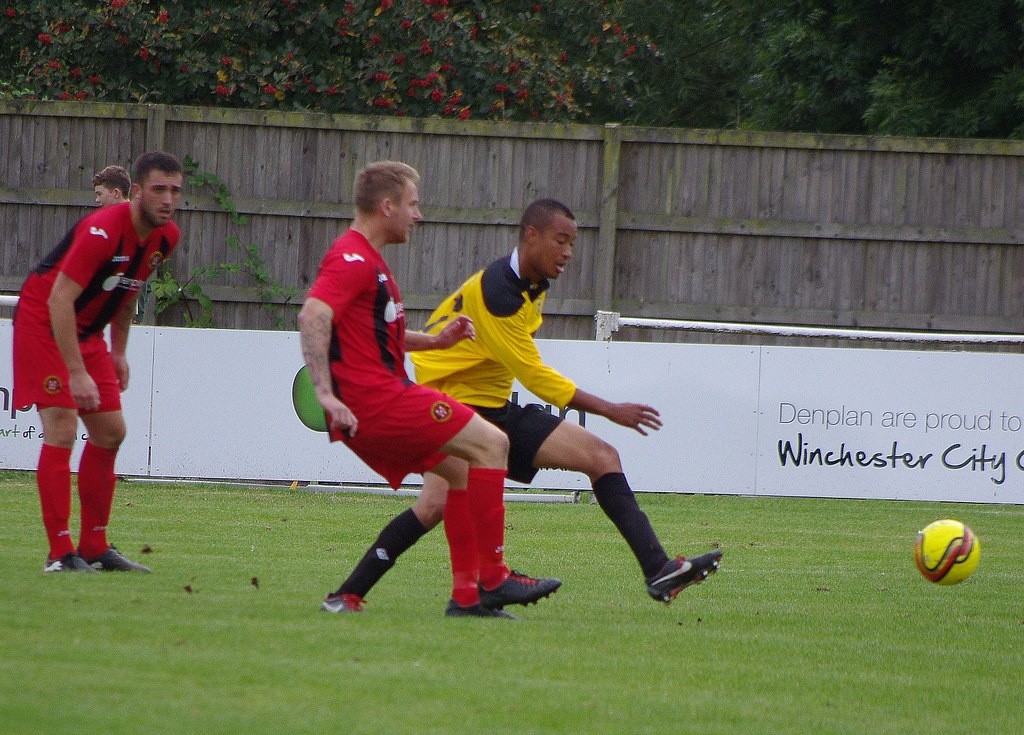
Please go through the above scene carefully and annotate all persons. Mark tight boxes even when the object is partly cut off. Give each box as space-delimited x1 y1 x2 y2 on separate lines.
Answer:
92 166 152 324
297 161 562 619
11 150 184 575
320 197 724 613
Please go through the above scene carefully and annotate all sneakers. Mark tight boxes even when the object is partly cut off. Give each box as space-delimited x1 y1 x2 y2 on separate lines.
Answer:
42 551 100 575
444 599 518 620
477 569 562 610
646 551 723 604
76 543 150 574
318 593 368 612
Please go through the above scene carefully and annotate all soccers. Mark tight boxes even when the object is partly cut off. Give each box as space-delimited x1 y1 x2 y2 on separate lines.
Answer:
914 520 982 587
